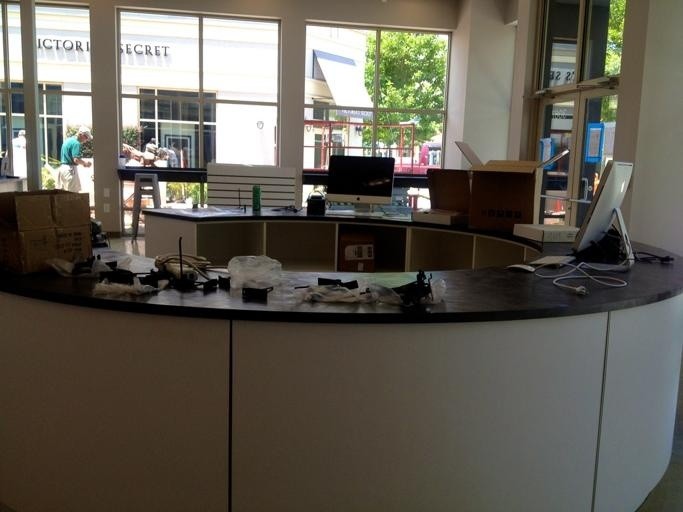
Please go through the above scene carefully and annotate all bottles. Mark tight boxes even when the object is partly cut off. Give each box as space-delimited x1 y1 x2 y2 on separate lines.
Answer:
252 185 260 211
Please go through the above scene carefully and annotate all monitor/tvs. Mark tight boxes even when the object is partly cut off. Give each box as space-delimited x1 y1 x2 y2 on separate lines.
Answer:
572 161 635 273
326 155 395 215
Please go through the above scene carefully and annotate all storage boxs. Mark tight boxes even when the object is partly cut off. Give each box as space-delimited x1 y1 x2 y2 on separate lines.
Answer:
0 189 91 275
454 141 569 229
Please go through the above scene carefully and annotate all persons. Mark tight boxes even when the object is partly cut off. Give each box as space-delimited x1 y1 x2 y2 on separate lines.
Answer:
58 126 93 193
12 129 28 181
137 136 178 169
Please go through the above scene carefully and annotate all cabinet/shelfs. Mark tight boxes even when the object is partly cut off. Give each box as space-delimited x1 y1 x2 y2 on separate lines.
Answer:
197 219 540 272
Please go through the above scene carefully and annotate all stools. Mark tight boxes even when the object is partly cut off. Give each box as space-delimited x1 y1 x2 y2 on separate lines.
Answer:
130 174 160 243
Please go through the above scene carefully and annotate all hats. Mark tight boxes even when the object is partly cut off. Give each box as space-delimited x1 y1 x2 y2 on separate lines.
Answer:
79 126 93 139
